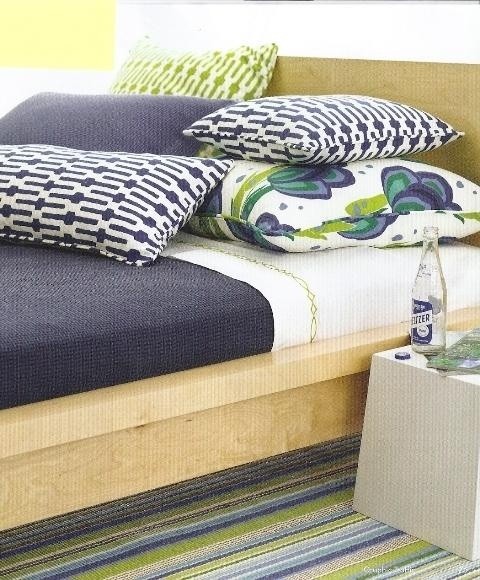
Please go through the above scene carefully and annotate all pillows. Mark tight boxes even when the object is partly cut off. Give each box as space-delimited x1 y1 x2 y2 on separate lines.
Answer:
0 30 479 266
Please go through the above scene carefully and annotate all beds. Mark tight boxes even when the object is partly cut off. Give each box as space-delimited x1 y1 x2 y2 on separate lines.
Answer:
0 56 479 535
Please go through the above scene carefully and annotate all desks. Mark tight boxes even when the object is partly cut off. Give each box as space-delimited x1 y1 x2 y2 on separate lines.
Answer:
351 331 479 560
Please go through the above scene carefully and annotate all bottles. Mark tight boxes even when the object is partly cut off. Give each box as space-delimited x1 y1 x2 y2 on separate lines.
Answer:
410 228 448 354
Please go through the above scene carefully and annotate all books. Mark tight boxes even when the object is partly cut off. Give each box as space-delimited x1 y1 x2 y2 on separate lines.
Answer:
425 327 480 377
